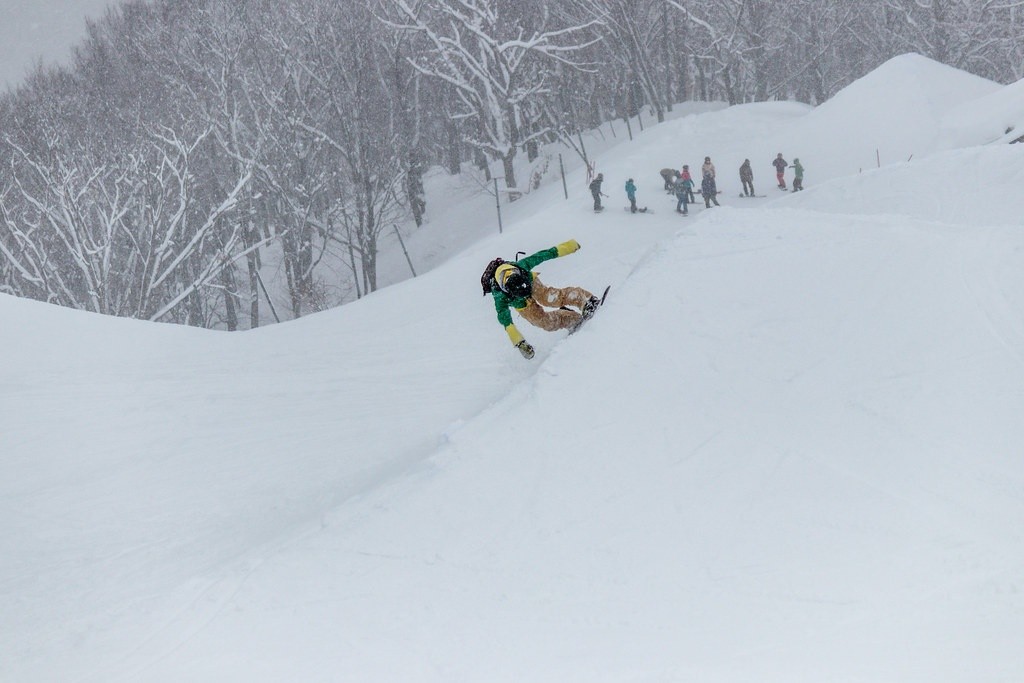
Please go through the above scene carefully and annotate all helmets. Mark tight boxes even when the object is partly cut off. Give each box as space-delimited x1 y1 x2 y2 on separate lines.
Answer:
507 275 532 297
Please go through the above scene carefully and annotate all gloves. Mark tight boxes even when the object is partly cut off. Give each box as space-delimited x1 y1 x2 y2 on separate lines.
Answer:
517 340 535 359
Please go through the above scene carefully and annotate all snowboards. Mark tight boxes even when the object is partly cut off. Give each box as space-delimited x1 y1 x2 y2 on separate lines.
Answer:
628 205 648 213
594 205 604 214
740 192 768 197
566 281 613 337
672 199 703 204
790 188 805 192
699 189 723 193
678 190 700 194
776 181 790 191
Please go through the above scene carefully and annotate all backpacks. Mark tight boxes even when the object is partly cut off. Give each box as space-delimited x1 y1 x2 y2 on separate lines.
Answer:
481 257 526 297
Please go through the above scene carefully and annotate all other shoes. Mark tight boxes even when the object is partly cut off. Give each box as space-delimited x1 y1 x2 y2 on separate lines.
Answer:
566 314 583 333
583 295 600 320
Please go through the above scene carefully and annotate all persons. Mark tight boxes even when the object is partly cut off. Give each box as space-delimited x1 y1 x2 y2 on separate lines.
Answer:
591 173 605 213
739 159 756 197
772 153 788 189
661 157 720 216
625 178 638 213
789 158 804 192
489 239 599 359
